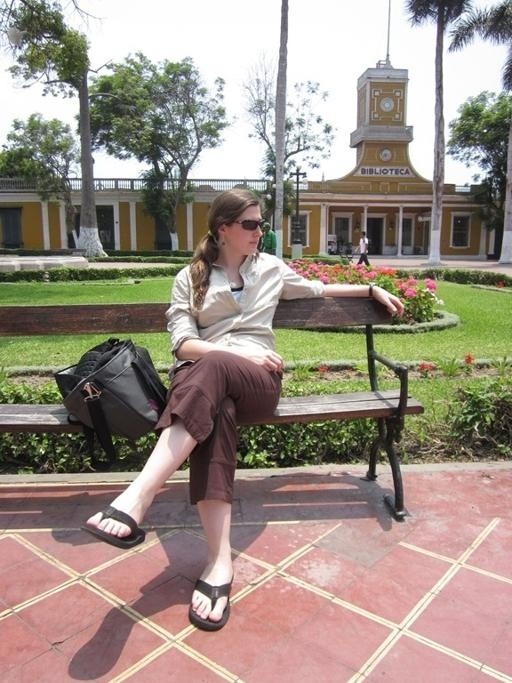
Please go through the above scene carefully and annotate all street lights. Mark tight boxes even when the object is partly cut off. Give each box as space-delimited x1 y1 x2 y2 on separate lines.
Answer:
289 166 307 244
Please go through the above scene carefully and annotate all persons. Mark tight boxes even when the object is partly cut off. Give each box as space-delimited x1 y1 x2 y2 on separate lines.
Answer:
79 188 407 633
260 221 278 255
354 230 371 263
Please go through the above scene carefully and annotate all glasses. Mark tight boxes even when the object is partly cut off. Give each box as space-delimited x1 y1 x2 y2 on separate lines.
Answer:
231 217 265 232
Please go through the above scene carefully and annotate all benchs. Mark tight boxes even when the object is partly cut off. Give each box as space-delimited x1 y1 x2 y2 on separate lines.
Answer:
1 297 424 522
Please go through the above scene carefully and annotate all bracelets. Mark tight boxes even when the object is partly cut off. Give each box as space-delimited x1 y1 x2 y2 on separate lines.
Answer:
368 280 376 298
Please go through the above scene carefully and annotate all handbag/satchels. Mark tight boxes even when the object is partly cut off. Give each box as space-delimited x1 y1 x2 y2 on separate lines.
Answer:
53 337 170 471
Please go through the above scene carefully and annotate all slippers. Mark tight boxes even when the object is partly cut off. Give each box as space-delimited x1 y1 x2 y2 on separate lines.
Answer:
188 569 234 633
77 504 146 551
